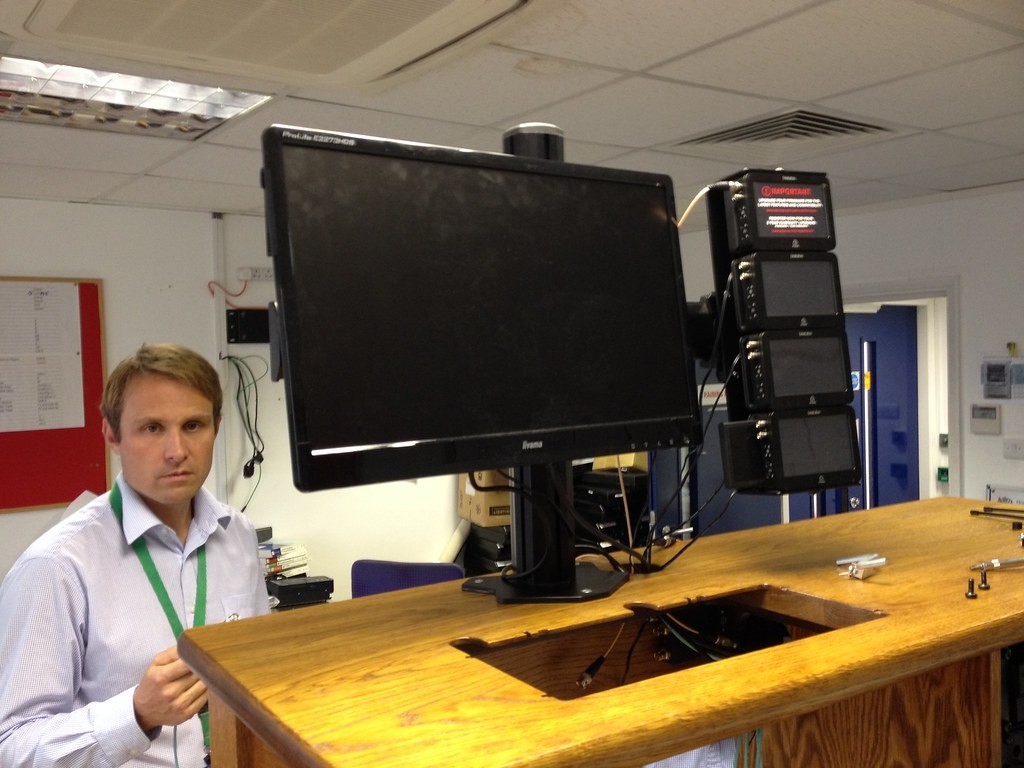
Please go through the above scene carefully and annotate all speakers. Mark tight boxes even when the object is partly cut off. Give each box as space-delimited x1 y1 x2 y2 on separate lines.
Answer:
710 169 863 496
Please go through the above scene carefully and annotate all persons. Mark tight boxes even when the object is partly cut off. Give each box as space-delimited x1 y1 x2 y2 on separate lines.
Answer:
1 341 273 768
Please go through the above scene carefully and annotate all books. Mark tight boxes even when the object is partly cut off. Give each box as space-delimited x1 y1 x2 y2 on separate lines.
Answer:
258 543 311 582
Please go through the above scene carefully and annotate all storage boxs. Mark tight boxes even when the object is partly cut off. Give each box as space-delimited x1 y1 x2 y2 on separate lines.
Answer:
456 468 512 527
591 453 650 474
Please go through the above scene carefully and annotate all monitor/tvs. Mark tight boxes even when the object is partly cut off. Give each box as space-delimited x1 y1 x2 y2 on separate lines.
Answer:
261 123 703 604
